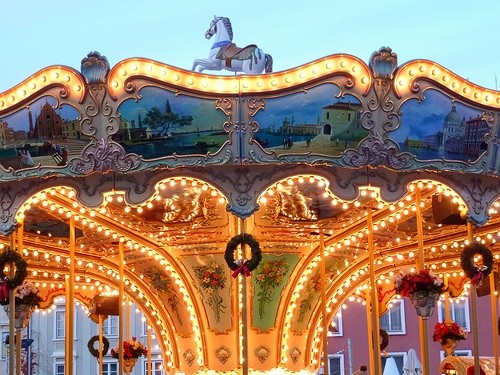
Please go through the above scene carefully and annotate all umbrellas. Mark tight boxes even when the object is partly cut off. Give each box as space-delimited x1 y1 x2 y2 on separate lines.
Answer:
403 348 422 375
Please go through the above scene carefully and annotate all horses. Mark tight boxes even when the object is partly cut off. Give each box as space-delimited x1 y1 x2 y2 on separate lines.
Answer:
188 13 274 76
